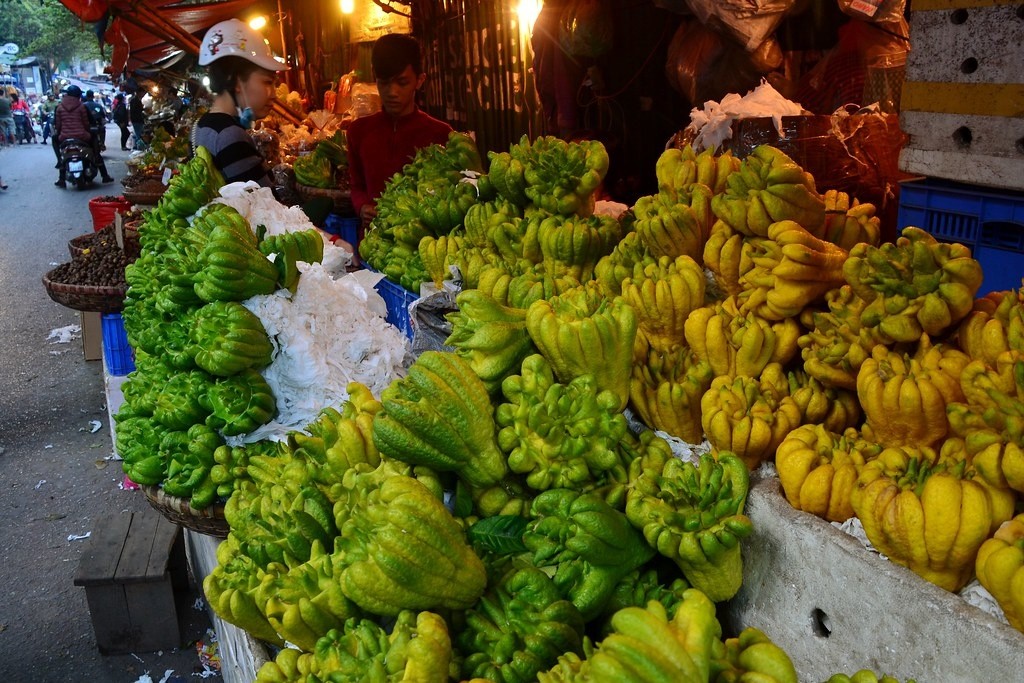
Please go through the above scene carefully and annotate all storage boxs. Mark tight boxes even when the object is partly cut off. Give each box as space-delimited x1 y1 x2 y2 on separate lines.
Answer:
81 310 139 460
894 0 1024 298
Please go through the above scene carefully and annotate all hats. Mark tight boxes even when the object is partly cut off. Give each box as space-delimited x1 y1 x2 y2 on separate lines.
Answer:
67 85 85 93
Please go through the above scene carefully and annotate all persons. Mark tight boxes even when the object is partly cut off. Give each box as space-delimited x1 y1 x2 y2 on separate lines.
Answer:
51 85 114 188
188 18 359 267
0 176 8 190
0 89 62 147
113 89 202 151
345 34 452 262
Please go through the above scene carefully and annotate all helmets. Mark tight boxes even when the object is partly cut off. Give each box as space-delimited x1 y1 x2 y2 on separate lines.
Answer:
47 92 54 97
199 18 292 70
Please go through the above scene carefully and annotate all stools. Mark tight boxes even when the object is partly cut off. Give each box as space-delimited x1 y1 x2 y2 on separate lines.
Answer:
74 507 183 656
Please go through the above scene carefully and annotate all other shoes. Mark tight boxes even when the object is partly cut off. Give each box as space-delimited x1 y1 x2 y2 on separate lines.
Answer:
55 180 66 188
0 185 8 189
122 147 131 151
102 175 114 183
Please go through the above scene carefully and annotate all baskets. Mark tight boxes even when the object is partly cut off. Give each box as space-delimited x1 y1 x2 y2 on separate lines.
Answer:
142 483 230 540
42 266 129 313
68 233 95 258
295 181 351 211
125 220 146 237
122 191 163 204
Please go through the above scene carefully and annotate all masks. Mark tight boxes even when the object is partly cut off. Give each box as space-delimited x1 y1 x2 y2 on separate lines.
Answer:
236 82 256 129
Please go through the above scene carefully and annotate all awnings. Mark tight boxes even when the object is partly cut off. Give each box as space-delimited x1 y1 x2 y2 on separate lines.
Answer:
57 0 275 85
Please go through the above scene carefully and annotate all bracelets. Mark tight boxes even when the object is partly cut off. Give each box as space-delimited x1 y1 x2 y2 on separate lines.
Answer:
329 235 340 245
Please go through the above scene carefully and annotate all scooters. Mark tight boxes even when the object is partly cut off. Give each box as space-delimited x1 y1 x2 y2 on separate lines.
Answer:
11 108 32 144
61 139 99 190
